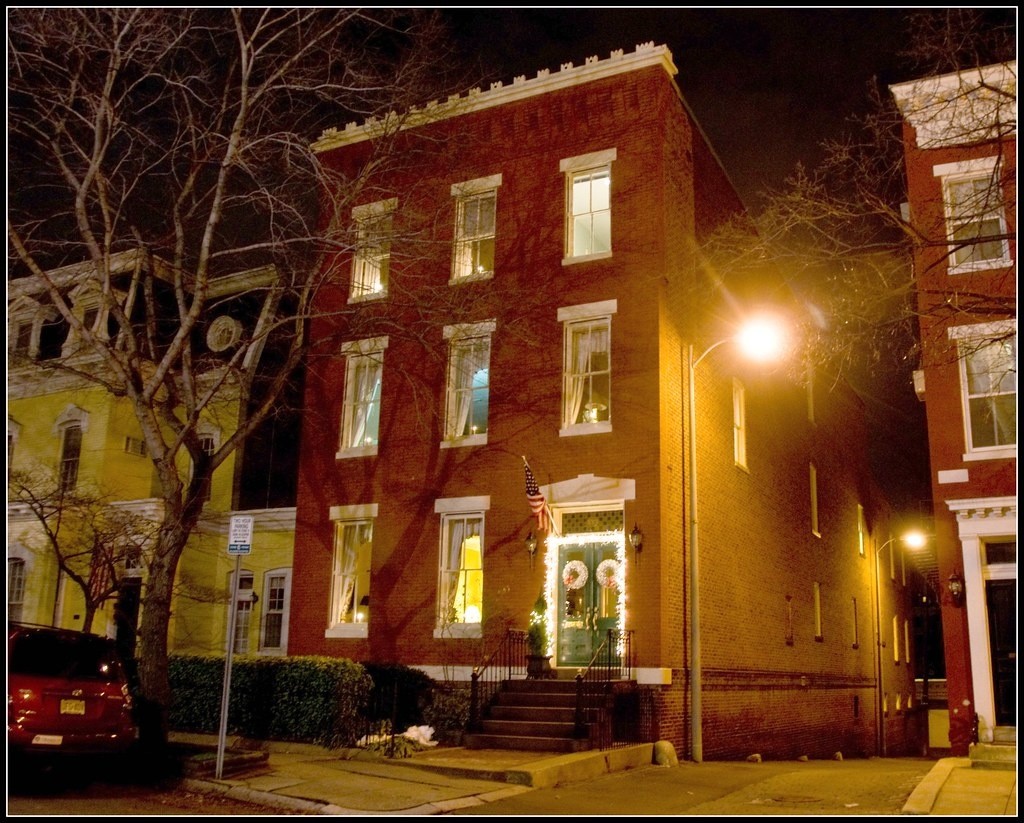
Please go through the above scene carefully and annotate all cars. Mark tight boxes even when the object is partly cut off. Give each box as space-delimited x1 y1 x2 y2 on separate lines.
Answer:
8 620 140 770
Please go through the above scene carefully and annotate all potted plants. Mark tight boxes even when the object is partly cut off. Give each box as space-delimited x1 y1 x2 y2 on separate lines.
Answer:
525 597 554 680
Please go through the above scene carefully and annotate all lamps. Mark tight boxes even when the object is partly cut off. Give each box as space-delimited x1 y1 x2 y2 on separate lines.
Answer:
948 569 963 605
628 522 643 554
524 526 538 555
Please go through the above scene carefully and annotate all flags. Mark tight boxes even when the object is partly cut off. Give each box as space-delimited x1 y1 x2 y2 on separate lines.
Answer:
524 463 549 533
87 538 112 610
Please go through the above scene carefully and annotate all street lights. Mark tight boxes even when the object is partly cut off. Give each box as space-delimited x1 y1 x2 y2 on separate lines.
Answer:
875 535 925 759
689 321 791 763
